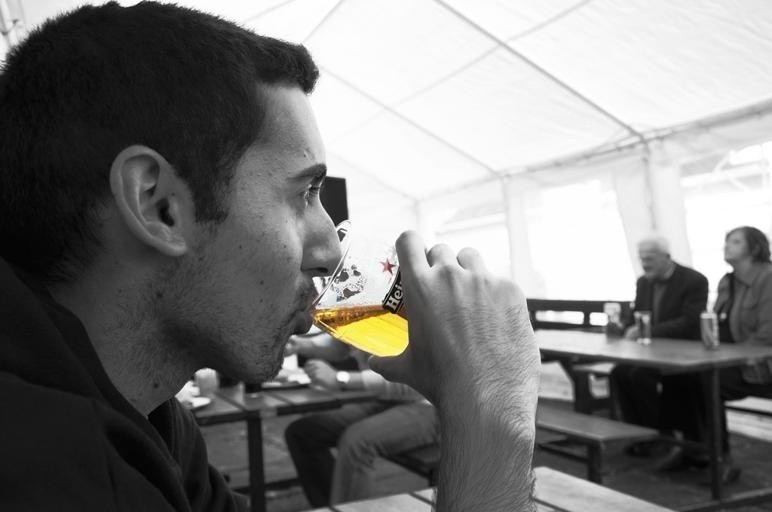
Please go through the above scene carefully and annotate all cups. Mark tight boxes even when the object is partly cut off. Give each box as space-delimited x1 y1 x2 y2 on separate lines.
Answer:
700 311 722 351
633 310 655 345
194 368 218 401
302 218 442 358
603 303 622 340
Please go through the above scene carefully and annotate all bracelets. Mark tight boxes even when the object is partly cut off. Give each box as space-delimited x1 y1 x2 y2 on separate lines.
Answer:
335 370 351 392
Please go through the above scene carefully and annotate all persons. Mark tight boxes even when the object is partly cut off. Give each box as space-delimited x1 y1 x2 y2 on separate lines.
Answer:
610 233 707 466
0 0 542 511
280 326 439 505
663 222 772 485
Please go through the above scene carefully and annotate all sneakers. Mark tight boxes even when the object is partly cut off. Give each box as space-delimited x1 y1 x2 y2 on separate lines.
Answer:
654 437 743 488
622 429 675 459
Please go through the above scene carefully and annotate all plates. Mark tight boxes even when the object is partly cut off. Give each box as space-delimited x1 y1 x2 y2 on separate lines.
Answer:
241 370 319 391
167 397 211 411
